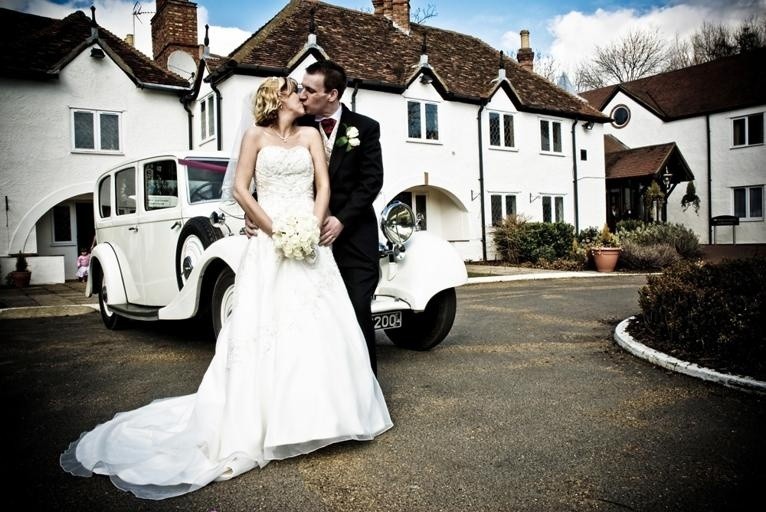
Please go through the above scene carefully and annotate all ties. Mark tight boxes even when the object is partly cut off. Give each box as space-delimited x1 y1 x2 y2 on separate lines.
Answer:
321 119 334 138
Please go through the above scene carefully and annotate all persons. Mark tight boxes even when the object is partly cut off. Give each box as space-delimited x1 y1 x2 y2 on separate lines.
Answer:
202 76 397 459
243 58 386 379
75 248 92 284
89 232 98 254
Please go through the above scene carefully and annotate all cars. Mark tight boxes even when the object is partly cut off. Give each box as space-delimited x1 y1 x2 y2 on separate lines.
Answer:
82 149 469 353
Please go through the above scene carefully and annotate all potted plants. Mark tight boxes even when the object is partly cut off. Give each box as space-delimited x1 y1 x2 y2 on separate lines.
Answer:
590 227 620 272
10 252 29 288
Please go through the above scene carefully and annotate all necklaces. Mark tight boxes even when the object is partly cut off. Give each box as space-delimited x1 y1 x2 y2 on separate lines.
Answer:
271 125 295 144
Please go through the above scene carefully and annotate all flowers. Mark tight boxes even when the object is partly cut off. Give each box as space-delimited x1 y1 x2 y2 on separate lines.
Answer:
336 124 360 152
270 214 321 267
